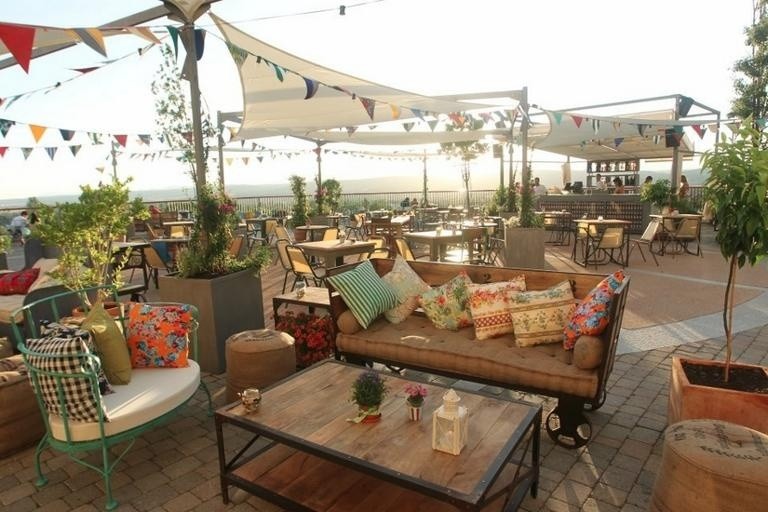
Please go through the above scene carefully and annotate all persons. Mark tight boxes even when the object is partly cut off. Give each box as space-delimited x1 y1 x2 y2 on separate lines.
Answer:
400 197 410 206
514 182 521 193
411 198 420 206
8 210 29 229
147 205 161 215
595 174 606 191
532 177 548 195
613 177 624 194
30 211 41 225
640 175 653 193
679 174 690 199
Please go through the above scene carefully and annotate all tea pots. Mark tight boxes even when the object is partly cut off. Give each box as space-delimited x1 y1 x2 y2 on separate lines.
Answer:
236 388 261 413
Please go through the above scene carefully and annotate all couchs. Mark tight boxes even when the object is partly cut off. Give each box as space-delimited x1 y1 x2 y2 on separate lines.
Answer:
324 256 632 450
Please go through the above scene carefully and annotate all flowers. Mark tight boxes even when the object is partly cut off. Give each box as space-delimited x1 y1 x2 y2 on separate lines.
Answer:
404 383 429 407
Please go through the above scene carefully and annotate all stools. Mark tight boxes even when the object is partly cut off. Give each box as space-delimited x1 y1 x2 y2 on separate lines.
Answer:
643 417 768 511
225 327 296 400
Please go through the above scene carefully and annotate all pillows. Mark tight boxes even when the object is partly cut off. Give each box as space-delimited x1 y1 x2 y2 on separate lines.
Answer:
1 266 43 298
328 258 624 353
25 300 192 423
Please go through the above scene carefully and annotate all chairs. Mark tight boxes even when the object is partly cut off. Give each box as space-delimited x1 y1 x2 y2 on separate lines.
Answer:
1 256 93 326
9 285 215 511
535 210 705 270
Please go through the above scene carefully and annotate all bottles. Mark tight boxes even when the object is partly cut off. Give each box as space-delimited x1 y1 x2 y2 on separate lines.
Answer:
431 387 468 456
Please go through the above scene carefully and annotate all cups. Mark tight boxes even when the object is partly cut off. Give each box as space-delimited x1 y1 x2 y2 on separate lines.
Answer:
294 282 305 297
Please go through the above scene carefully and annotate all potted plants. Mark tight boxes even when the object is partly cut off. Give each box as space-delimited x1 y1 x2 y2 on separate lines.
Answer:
667 115 768 431
487 141 544 271
348 370 392 425
158 183 273 377
24 175 152 319
286 176 341 244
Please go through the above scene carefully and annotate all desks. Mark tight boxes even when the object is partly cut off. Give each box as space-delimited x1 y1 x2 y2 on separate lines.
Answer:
272 285 333 347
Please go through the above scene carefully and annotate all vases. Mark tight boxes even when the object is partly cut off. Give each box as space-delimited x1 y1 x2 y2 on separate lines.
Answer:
407 407 423 419
